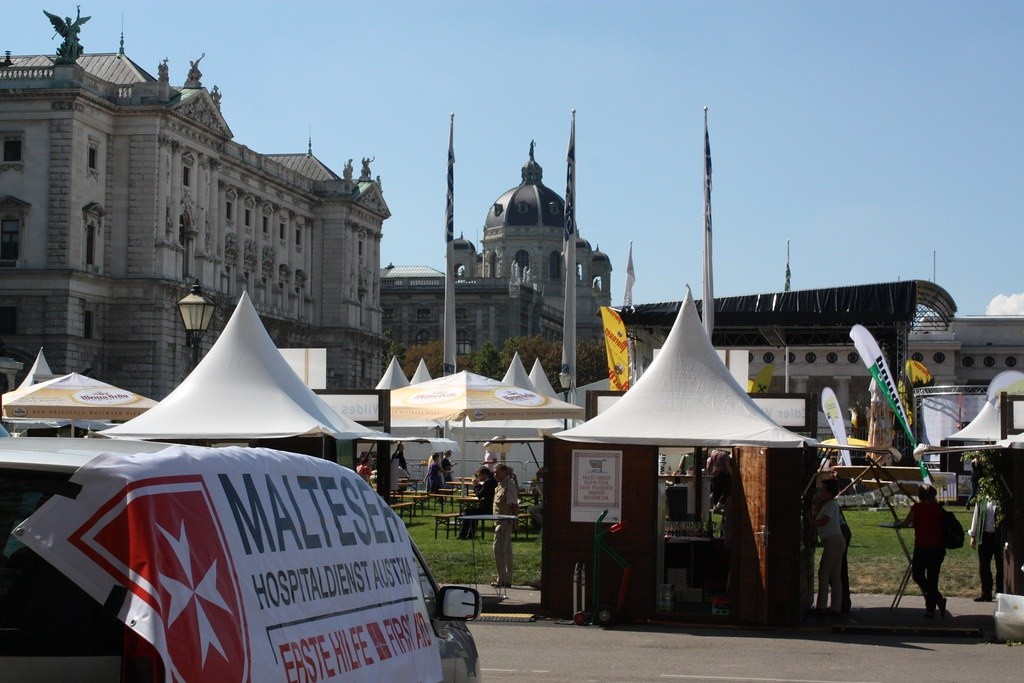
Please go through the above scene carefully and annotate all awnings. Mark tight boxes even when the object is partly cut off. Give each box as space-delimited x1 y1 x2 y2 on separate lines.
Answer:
357 436 461 474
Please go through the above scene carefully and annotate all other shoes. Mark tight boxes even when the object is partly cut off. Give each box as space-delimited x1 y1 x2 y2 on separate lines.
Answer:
973 596 991 602
492 582 512 587
925 610 936 616
940 599 947 615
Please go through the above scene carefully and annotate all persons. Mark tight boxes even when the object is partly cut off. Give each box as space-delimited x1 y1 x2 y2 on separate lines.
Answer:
807 479 851 615
968 494 1003 601
363 157 375 177
62 17 80 58
894 484 947 617
706 449 733 514
357 442 543 588
966 451 984 510
511 260 531 282
187 51 205 79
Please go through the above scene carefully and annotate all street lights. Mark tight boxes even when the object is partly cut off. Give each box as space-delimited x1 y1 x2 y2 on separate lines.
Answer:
558 371 572 430
175 278 218 371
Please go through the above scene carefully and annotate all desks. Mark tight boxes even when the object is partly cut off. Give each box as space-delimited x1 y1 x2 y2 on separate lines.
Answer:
390 483 407 519
522 481 542 507
460 513 519 599
400 479 422 515
445 481 473 514
665 538 727 590
878 524 917 610
455 476 473 496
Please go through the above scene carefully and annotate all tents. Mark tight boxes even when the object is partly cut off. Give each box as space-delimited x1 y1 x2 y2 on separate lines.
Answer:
542 285 818 624
946 402 1001 472
2 291 390 458
375 352 586 485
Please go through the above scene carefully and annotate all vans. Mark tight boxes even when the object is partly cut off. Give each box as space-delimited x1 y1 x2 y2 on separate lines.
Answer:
0 435 482 683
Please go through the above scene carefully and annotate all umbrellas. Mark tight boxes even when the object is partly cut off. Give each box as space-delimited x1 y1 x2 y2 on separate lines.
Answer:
821 436 868 451
2 372 160 437
390 370 585 497
865 379 893 466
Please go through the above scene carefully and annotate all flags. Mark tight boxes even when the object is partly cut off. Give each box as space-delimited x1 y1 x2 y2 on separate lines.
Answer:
751 366 775 392
850 324 932 485
601 306 629 391
623 244 635 305
822 388 855 488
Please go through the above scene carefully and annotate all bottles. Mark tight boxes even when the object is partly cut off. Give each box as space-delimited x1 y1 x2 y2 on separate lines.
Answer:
720 512 725 537
707 512 713 537
660 584 673 610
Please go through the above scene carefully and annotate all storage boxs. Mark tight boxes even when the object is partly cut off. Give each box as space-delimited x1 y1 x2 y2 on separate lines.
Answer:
667 568 704 603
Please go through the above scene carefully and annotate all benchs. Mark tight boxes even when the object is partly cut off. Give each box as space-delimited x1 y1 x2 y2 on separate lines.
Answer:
390 489 541 540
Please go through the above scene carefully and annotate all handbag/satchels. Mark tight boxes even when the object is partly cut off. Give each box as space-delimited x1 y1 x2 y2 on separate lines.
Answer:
944 510 965 549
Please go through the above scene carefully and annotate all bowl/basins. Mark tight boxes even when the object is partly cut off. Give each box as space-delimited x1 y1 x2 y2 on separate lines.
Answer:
473 481 479 486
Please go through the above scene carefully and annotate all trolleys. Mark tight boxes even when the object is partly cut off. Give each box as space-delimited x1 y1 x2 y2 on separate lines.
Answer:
592 508 631 626
